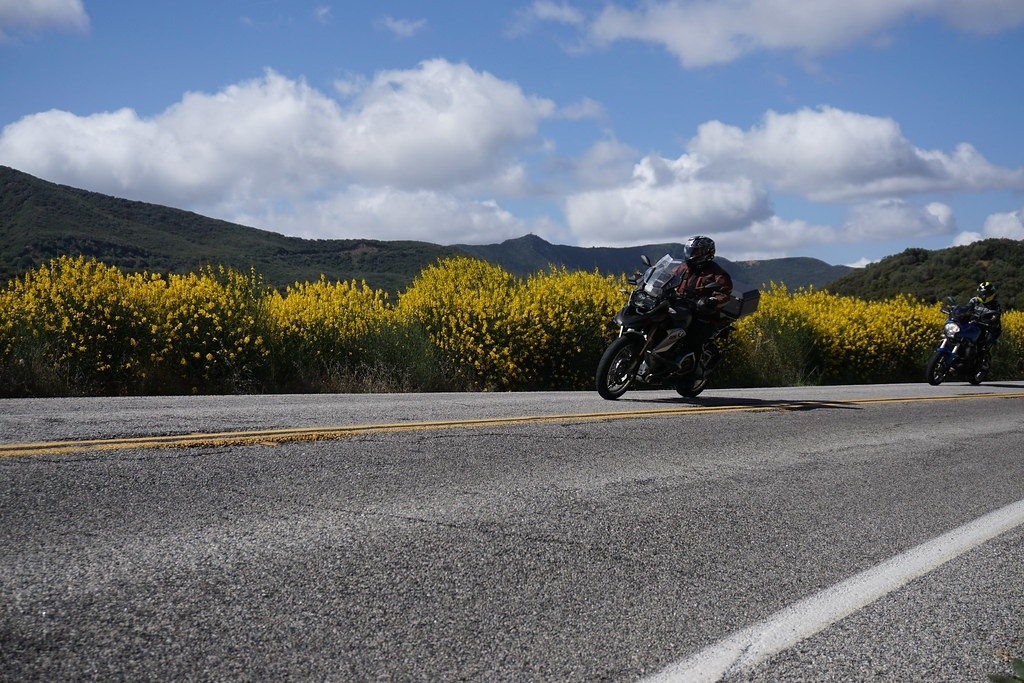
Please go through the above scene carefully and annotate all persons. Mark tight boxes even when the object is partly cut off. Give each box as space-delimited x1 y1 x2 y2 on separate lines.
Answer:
964 281 1002 366
666 235 733 364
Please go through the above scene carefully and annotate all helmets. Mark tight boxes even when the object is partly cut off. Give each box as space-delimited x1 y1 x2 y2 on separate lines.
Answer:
683 235 715 268
977 282 996 303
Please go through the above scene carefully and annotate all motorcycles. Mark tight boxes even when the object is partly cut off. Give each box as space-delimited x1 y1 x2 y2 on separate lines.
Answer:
927 297 992 386
596 254 760 400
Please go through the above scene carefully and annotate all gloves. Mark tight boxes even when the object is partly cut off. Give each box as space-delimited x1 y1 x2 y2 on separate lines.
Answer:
697 296 719 310
